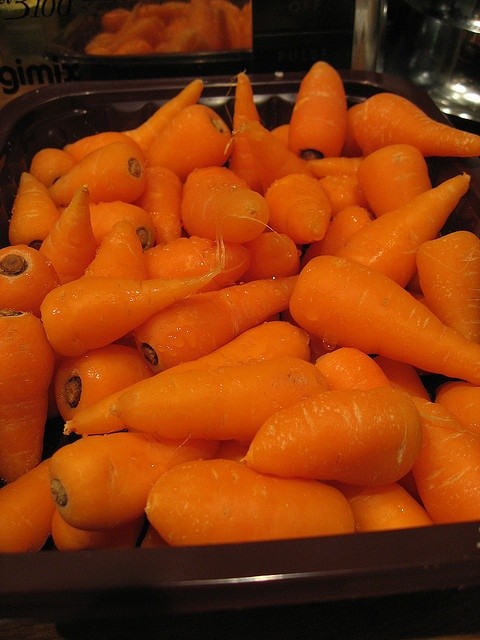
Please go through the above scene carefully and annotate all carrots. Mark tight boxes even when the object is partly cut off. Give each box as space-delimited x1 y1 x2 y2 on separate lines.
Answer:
0 62 478 555
84 2 253 55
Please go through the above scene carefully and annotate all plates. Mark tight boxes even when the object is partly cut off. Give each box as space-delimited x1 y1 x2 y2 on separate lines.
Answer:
56 0 299 80
0 70 480 625
